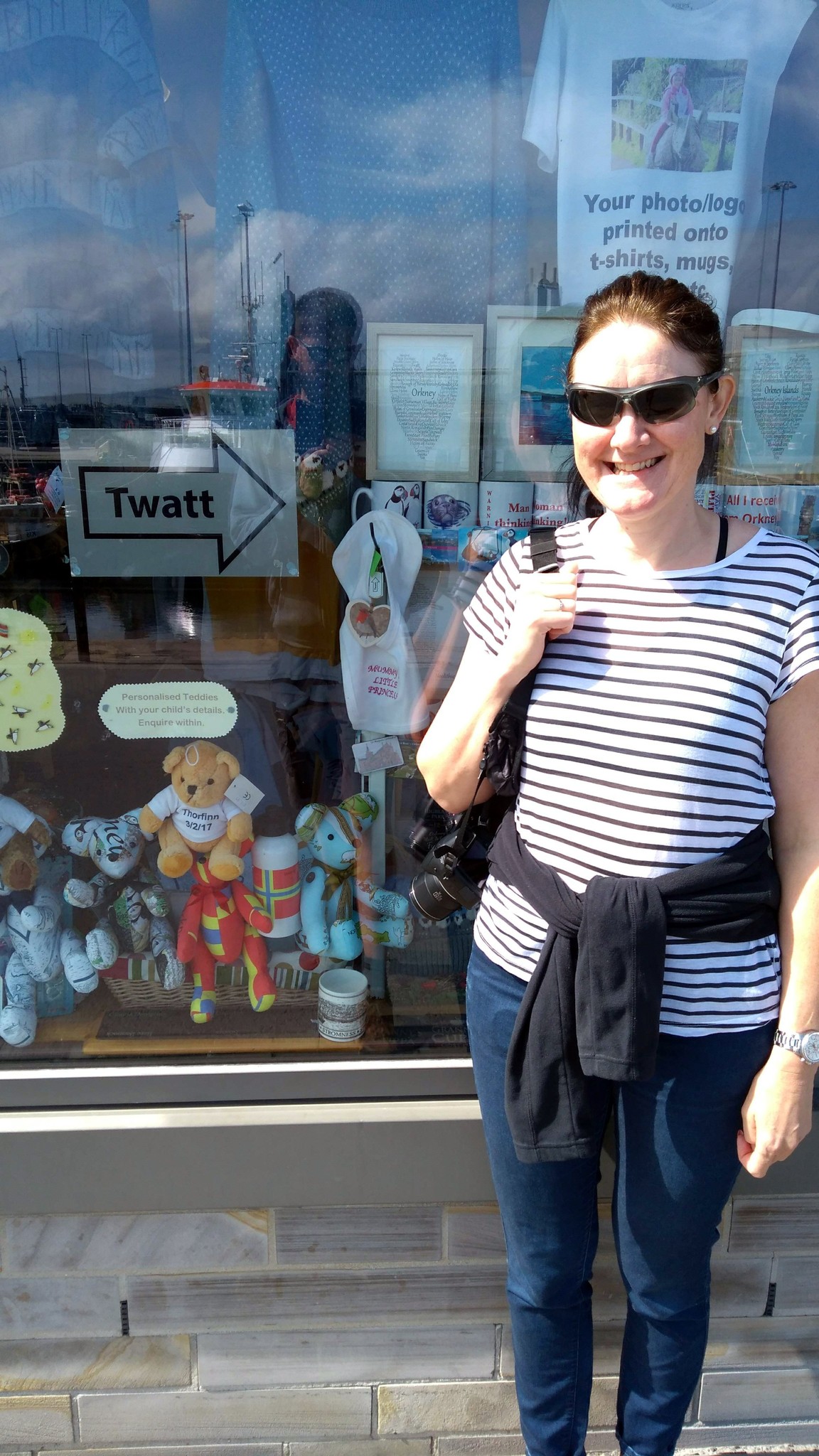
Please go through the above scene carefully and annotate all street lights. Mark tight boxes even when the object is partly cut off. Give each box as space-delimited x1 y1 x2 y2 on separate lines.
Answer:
236 199 259 381
50 327 63 403
81 334 93 407
763 178 796 341
175 213 198 385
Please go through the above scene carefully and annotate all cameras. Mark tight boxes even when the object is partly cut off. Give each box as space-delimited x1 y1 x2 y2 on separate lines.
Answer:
408 807 502 922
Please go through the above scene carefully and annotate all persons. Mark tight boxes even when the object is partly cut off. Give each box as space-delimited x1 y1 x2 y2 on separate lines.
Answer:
409 251 819 1456
650 64 705 167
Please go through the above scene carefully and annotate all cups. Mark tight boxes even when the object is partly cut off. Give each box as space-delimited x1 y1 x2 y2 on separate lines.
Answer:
424 481 478 530
478 480 534 531
778 485 819 549
723 484 780 534
351 480 423 529
693 486 724 517
317 965 368 1043
532 481 581 528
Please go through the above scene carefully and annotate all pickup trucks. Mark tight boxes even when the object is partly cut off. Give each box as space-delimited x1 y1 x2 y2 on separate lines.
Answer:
0 409 71 444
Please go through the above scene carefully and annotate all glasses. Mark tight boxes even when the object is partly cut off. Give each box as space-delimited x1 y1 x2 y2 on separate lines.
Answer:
566 366 731 427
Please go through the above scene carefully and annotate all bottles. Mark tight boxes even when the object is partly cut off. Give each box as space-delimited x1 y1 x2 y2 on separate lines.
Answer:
250 802 302 938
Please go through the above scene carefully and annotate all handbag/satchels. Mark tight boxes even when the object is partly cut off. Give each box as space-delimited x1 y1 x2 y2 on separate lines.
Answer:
405 524 556 920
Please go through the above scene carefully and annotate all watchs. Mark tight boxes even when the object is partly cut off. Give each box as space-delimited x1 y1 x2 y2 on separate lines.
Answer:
773 1028 819 1065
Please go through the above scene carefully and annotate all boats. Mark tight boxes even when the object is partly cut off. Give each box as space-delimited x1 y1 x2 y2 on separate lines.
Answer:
153 342 278 475
0 367 66 545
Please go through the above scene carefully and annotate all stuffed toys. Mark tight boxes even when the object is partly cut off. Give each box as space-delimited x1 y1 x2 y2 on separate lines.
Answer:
0 740 412 1050
